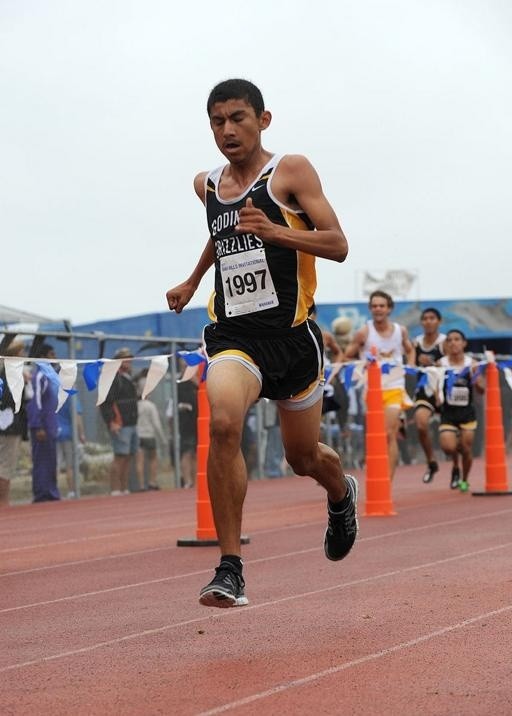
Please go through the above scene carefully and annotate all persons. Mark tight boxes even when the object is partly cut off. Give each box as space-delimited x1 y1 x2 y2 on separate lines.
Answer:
172 360 199 491
309 292 485 492
0 342 85 504
166 78 360 610
241 397 283 479
99 348 164 493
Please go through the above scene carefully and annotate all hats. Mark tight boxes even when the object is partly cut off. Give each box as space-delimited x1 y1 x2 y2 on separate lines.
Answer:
113 347 133 359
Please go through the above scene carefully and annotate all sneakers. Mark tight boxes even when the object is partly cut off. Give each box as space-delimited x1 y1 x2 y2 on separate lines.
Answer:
324 476 358 562
198 563 249 609
423 462 469 491
